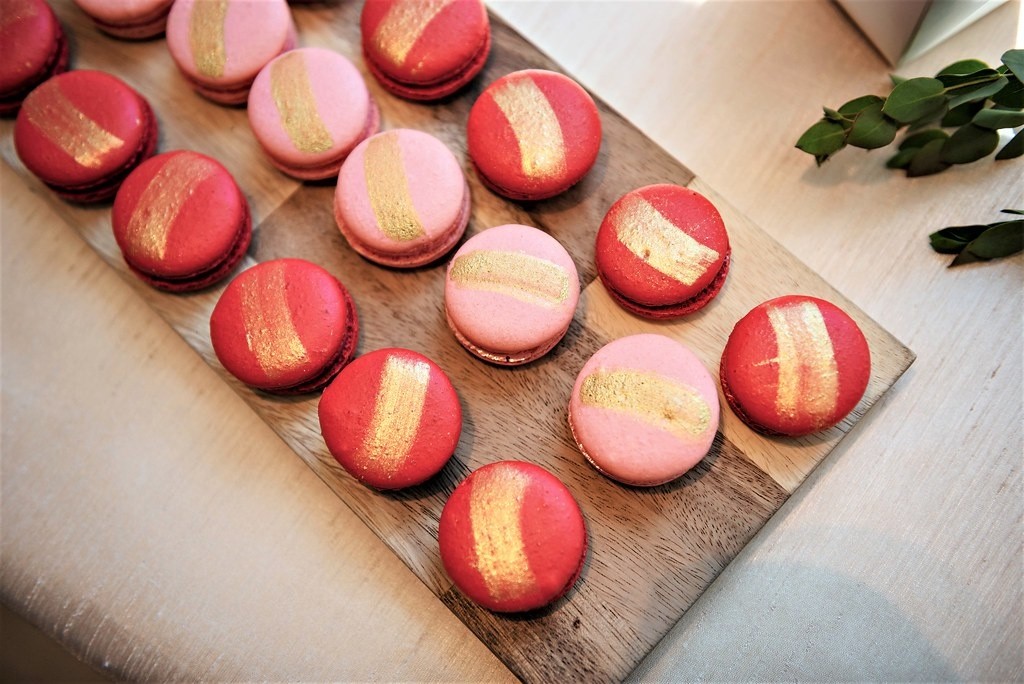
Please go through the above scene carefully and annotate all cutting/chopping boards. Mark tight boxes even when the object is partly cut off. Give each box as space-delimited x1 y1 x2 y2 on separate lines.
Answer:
1 1 915 683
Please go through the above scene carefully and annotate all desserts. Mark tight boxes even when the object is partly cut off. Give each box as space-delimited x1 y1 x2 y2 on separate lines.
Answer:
0 0 872 615
